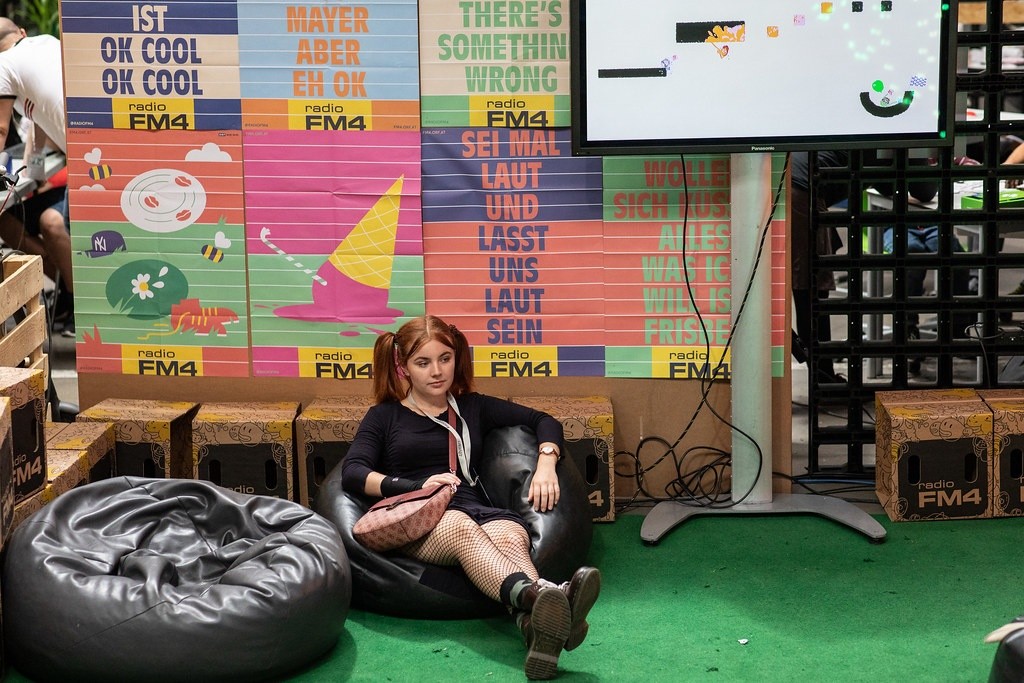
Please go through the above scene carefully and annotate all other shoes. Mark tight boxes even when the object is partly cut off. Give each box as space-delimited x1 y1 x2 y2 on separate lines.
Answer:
62 313 75 336
51 294 70 320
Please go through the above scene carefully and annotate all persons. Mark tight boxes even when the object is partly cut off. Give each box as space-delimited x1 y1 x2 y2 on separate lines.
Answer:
789 151 939 405
883 157 979 377
340 315 601 681
0 18 70 234
0 111 75 338
1002 143 1024 165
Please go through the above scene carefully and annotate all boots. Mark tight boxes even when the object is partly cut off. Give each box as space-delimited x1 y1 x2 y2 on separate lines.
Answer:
521 565 602 651
518 587 571 681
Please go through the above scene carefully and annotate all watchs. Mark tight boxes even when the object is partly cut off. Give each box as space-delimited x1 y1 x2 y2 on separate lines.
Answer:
539 446 560 460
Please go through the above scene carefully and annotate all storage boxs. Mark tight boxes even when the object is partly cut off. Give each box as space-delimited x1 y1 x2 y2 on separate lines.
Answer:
0 396 16 550
975 389 1024 517
512 394 615 522
14 484 55 525
961 187 1024 209
192 401 302 502
496 395 509 401
48 450 88 496
46 421 117 484
75 397 200 480
0 367 47 505
875 388 993 522
295 396 377 511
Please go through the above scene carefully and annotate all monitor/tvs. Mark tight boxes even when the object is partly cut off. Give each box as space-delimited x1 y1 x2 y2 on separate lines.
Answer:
568 0 959 156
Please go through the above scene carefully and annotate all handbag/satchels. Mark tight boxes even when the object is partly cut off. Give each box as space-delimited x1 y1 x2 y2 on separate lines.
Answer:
352 482 458 554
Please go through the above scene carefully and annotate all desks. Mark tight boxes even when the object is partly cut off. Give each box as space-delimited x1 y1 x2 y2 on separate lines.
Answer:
0 152 66 216
866 179 1024 379
